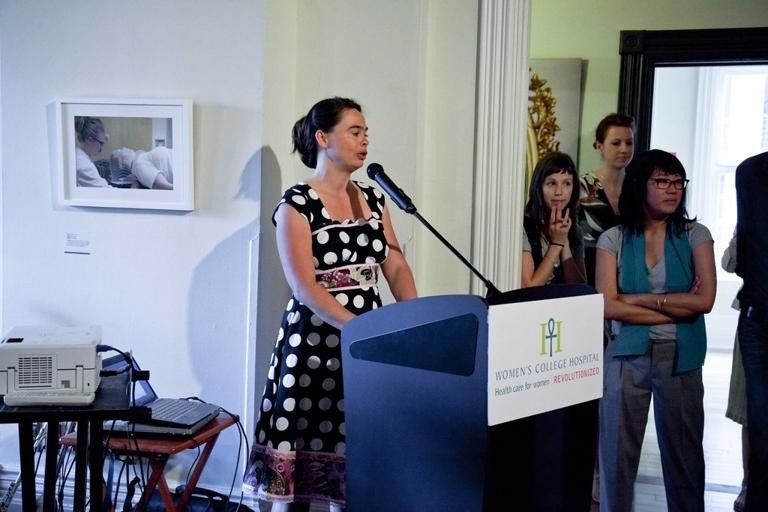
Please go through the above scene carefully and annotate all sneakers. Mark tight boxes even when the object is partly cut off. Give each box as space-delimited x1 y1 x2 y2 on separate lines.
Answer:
734 479 748 512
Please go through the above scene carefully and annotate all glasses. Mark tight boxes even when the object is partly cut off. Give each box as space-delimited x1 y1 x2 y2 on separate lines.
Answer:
93 137 103 145
647 178 689 190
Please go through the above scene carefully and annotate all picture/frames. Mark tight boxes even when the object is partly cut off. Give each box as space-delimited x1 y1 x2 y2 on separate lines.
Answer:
56 97 197 213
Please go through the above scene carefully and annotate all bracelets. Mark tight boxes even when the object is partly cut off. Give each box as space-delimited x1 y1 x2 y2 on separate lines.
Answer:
655 294 661 310
550 240 565 250
662 293 669 308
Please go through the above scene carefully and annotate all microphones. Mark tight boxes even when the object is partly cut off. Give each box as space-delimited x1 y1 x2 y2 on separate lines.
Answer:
367 162 417 215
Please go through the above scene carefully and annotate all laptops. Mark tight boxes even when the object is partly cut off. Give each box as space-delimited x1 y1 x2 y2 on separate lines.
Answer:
102 353 221 427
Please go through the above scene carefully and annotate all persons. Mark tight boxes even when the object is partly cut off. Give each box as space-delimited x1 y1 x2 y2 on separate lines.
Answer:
735 152 767 512
239 96 419 512
109 145 173 189
576 109 637 505
720 226 746 511
592 149 717 512
521 151 587 288
74 115 120 188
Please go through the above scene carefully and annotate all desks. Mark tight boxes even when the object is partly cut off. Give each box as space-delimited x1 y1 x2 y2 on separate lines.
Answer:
0 361 238 512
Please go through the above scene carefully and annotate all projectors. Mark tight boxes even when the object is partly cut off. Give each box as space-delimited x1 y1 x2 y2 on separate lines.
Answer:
0 324 102 407
